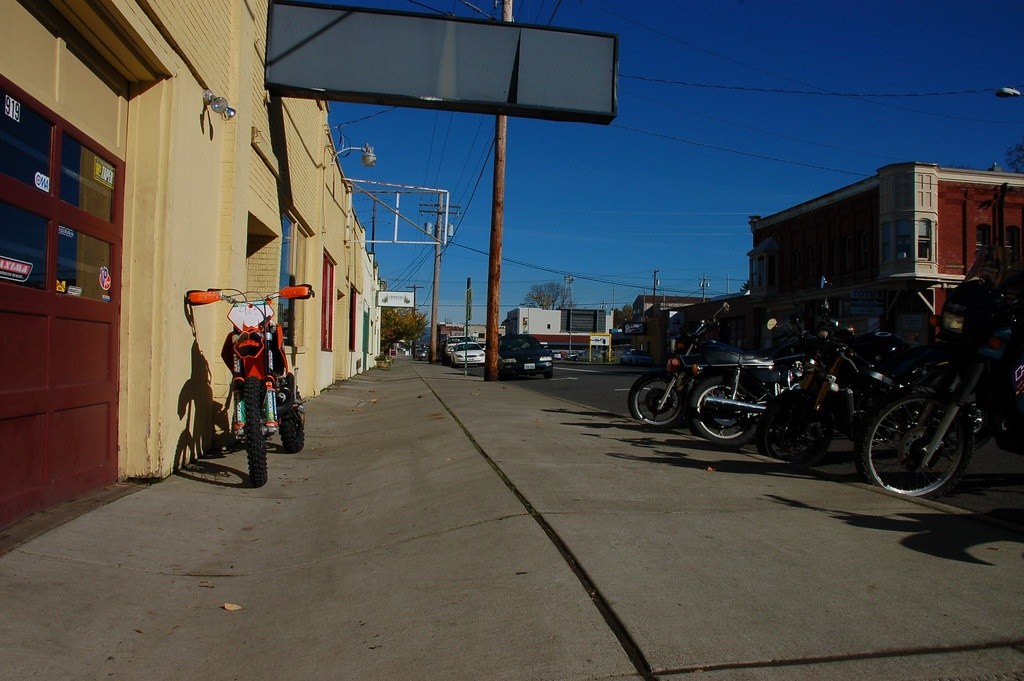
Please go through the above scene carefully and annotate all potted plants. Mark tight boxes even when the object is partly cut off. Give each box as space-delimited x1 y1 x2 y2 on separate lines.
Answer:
374 353 386 369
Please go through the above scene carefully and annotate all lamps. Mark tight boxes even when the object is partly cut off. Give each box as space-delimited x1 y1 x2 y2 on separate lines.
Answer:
203 90 237 121
330 143 377 167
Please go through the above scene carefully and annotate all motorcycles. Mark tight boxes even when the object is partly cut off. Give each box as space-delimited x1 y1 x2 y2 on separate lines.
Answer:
186 283 316 489
626 223 1024 502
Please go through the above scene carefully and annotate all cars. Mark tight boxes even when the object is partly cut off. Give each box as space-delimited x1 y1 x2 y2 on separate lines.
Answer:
560 350 609 363
620 347 654 367
397 334 476 366
496 333 554 382
551 350 562 360
451 342 486 368
